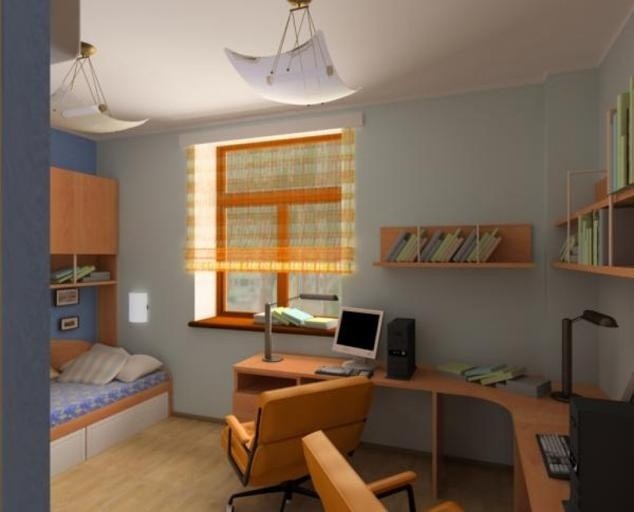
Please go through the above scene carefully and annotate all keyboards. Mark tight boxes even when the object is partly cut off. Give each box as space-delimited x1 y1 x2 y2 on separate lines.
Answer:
537 432 571 479
316 364 361 376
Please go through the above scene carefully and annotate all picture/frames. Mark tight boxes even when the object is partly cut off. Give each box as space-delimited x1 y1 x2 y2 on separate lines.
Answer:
54 287 80 307
60 315 80 331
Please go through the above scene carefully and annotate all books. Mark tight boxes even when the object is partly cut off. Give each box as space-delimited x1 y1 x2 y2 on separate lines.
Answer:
305 316 339 330
386 227 502 264
76 265 111 283
577 206 634 266
253 306 313 327
435 362 552 398
50 267 74 285
604 75 634 195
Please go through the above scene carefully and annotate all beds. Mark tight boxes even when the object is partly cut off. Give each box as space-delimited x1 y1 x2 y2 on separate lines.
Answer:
49 368 173 477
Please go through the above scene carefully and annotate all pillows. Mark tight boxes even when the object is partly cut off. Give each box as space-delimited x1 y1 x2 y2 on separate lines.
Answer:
49 340 91 371
49 365 59 380
60 343 129 372
115 354 162 383
57 350 127 385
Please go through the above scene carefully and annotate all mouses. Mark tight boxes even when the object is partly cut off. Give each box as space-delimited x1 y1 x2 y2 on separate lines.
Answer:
359 368 374 379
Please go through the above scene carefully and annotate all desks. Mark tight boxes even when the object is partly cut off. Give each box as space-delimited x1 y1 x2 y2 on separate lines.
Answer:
230 353 611 512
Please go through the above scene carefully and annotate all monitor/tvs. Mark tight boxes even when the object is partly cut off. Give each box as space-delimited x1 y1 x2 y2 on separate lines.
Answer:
562 392 634 512
331 308 384 369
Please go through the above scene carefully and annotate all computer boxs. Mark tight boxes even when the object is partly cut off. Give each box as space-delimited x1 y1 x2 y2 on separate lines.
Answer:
386 318 417 381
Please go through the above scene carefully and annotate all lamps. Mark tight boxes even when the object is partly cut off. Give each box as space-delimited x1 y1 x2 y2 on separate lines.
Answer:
552 310 619 403
263 293 339 363
50 41 148 134
225 0 361 105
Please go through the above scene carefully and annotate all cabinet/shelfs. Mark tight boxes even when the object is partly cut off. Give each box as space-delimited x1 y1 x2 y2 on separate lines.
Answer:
373 223 532 268
50 166 118 346
551 108 634 278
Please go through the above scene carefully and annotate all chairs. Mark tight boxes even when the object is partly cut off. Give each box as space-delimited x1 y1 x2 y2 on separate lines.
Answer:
302 430 464 512
218 375 373 512
